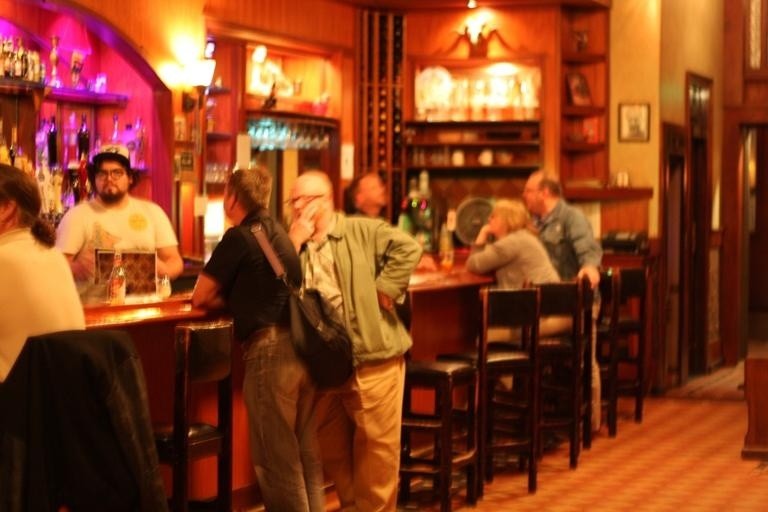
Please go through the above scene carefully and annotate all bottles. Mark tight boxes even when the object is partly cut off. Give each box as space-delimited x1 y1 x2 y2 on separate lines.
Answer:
0 110 145 172
1 37 47 82
105 241 126 308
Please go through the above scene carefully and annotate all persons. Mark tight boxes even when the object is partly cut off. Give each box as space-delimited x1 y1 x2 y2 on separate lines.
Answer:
189 165 354 512
288 169 424 512
465 197 574 350
56 141 185 309
520 167 606 431
0 165 89 382
347 171 388 217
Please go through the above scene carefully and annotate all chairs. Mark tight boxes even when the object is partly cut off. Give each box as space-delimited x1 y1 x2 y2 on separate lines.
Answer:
582 267 653 452
436 286 541 502
0 327 159 511
151 318 237 510
492 278 582 470
351 285 480 511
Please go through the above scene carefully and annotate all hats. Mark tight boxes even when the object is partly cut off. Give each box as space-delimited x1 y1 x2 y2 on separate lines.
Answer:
92 144 130 166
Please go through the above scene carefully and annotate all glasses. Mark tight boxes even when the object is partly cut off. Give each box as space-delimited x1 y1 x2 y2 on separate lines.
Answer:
284 193 324 204
520 188 543 193
98 170 122 179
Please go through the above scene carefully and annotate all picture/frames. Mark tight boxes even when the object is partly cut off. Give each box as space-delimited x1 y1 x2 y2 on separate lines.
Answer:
617 102 650 143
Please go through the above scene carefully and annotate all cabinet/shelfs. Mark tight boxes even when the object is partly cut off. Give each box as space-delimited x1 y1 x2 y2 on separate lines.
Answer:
45 86 147 225
406 53 549 205
0 78 45 172
560 0 610 187
208 39 236 197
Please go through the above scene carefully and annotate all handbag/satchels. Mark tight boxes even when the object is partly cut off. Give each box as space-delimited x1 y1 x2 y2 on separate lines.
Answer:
287 289 353 386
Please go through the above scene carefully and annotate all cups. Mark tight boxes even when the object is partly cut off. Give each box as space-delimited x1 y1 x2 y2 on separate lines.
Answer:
154 271 173 300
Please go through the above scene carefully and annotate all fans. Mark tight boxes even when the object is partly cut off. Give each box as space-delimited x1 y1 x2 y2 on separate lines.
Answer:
456 194 496 248
